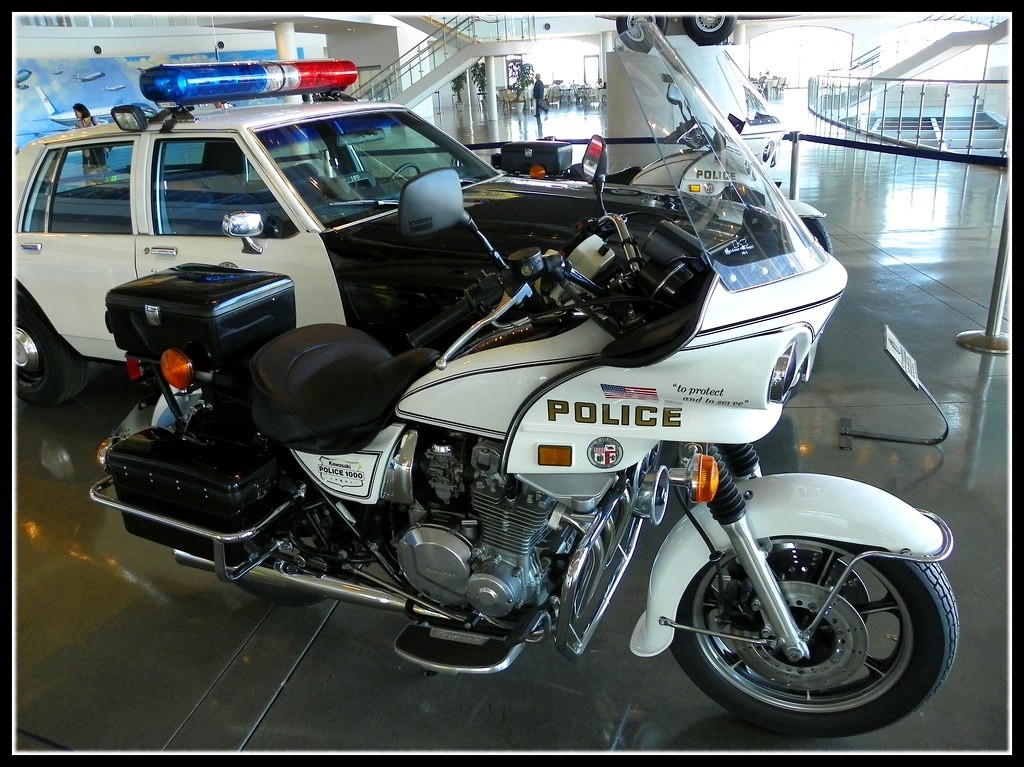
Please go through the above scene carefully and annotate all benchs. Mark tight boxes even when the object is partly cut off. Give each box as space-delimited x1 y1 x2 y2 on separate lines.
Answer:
161 167 245 197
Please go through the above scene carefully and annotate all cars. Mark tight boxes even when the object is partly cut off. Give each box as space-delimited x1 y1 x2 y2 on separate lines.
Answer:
14 59 720 406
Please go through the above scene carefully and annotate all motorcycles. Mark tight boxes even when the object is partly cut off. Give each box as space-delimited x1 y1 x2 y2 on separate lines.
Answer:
89 21 962 736
490 81 831 255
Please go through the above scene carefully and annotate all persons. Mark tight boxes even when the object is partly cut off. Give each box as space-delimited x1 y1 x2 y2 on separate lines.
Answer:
73 104 107 176
533 74 548 116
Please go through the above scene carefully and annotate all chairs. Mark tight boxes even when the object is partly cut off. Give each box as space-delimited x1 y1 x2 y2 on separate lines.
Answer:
279 161 335 205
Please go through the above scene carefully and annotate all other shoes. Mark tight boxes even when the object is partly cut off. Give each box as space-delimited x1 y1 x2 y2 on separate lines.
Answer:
545 109 548 114
534 115 540 117
